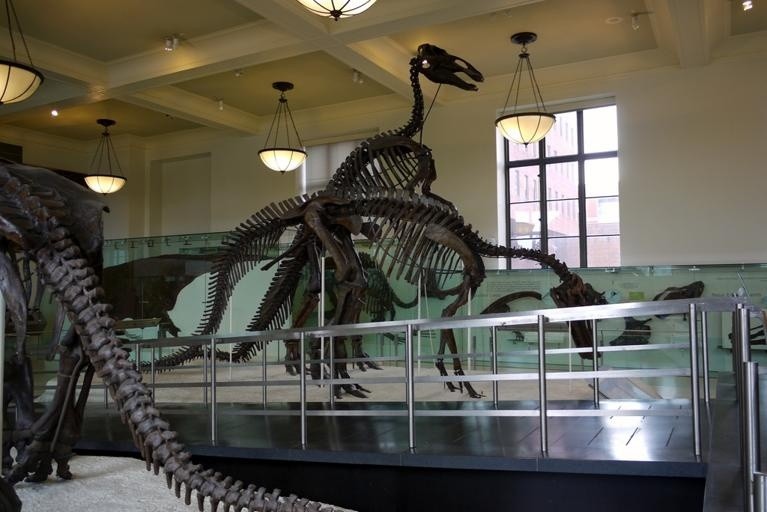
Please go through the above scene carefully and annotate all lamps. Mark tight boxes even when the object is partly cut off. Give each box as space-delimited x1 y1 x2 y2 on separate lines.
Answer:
0 19 47 108
83 116 128 198
493 30 557 149
163 36 180 54
257 81 309 176
295 0 379 26
